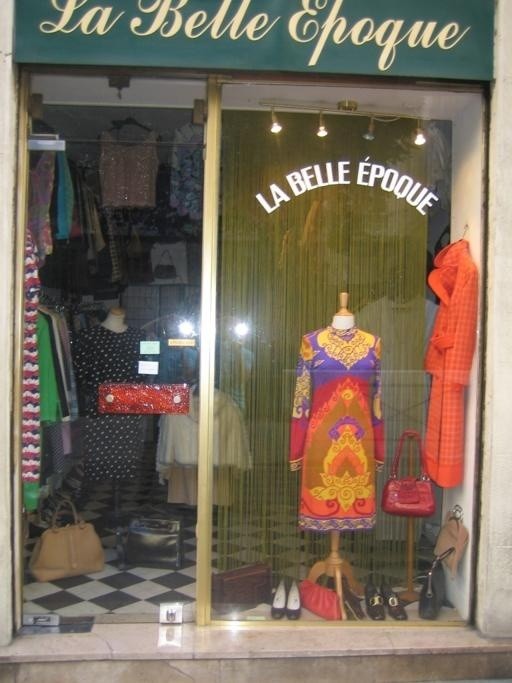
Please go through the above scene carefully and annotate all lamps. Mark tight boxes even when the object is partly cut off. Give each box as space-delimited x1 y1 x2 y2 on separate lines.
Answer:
259 101 431 145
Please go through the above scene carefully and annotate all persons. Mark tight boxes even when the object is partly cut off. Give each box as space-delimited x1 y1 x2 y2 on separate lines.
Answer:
74 308 152 484
288 310 387 539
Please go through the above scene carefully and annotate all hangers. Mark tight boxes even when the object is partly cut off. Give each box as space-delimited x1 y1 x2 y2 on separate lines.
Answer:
35 290 106 324
96 108 165 143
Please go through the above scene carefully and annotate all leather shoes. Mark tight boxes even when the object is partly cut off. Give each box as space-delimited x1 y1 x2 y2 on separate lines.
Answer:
381 583 407 620
286 579 301 620
365 583 385 621
272 580 286 619
327 577 366 620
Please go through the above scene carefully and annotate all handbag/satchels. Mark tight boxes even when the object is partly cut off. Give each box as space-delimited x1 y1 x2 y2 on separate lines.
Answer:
27 501 104 583
433 505 467 576
299 580 341 620
381 430 436 517
124 519 180 569
212 563 273 613
155 250 176 278
419 547 455 620
129 263 152 286
125 225 144 261
